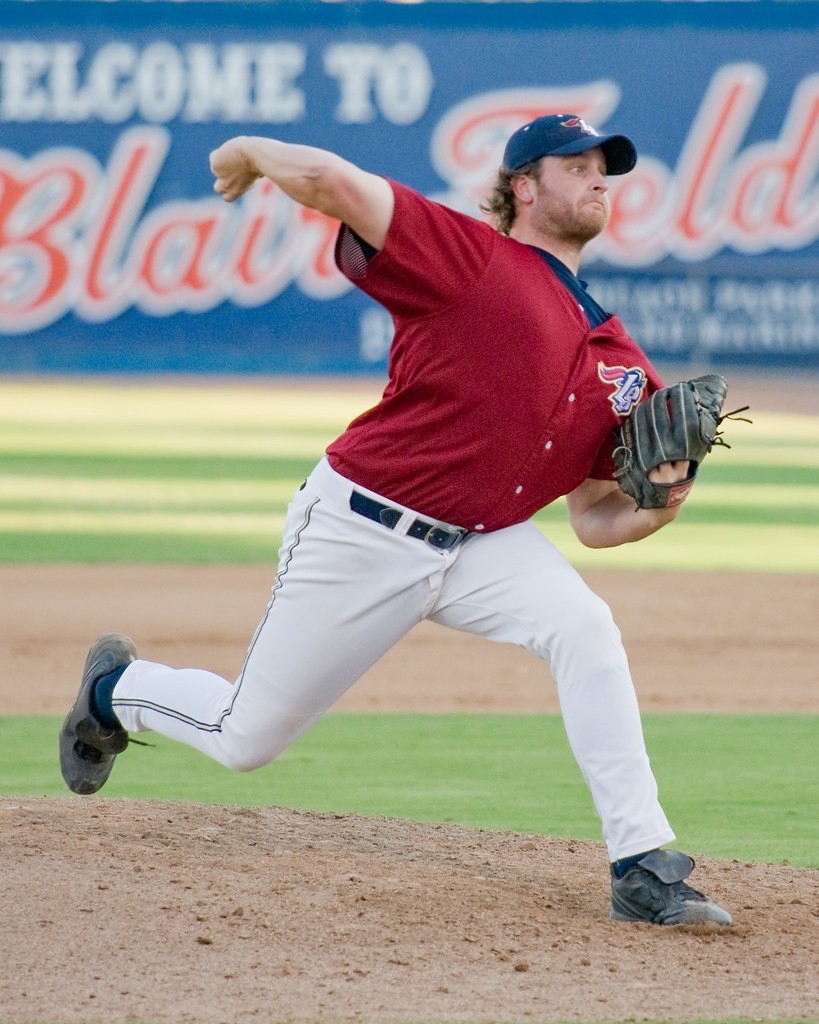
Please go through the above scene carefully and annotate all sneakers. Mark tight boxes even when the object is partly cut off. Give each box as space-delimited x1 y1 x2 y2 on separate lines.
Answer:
60 632 140 795
609 845 732 928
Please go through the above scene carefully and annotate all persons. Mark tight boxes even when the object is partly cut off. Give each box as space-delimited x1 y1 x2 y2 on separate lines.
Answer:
58 112 740 931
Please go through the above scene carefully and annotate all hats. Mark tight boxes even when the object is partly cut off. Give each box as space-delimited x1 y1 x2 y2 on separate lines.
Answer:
502 114 637 176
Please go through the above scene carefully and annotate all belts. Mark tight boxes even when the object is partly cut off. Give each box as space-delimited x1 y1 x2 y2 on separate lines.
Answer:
350 492 469 550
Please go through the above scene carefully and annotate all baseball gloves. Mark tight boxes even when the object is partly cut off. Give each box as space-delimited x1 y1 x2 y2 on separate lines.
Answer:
611 372 729 510
